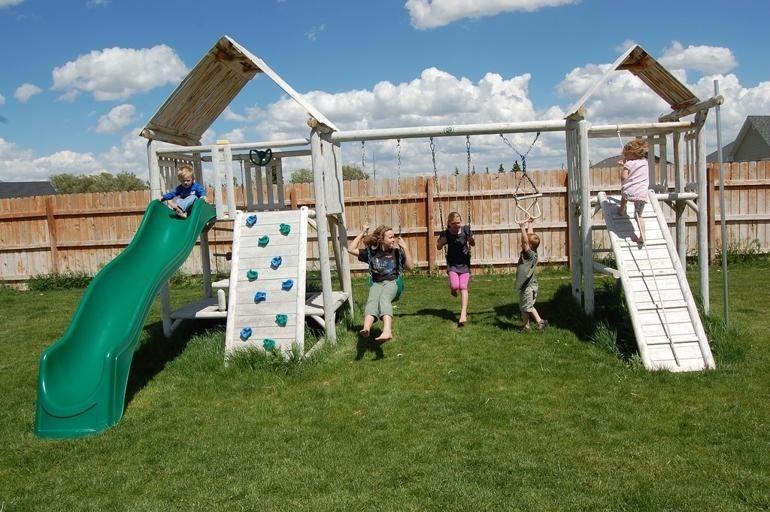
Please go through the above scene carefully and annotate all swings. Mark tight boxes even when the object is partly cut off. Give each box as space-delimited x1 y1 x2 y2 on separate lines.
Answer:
498 130 543 225
361 141 404 301
430 137 472 276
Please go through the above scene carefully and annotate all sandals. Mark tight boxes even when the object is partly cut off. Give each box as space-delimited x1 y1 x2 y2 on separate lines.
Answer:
516 319 548 333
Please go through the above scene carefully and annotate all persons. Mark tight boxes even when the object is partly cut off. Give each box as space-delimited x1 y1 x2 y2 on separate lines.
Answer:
515 217 549 333
347 222 414 342
618 139 651 245
156 163 210 218
436 212 476 325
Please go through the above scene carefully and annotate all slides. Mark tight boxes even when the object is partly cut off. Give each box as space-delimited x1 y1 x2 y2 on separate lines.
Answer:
35 198 215 440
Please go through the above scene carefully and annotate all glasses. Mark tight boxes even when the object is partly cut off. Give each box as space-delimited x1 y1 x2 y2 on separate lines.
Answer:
451 222 460 225
